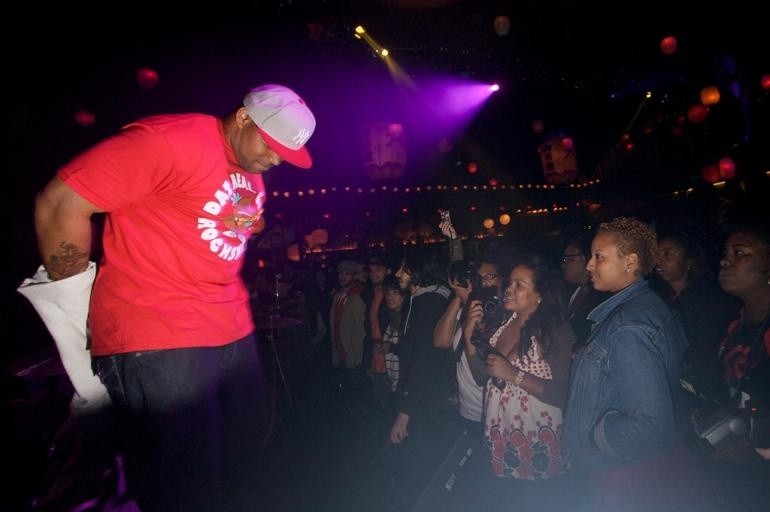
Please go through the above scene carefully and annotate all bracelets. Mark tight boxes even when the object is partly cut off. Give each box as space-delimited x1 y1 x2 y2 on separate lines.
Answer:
512 369 525 387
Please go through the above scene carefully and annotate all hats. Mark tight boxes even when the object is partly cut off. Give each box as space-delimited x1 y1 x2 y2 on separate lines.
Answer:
336 259 359 275
244 84 317 171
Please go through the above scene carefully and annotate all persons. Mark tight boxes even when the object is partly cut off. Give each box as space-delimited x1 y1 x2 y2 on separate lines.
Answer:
558 233 608 360
562 219 681 473
692 229 770 461
33 84 317 512
654 228 737 352
311 219 463 445
464 261 571 481
432 259 507 451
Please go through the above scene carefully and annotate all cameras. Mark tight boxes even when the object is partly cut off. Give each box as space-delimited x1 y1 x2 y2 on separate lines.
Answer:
440 210 450 223
482 296 502 323
446 259 479 284
677 409 747 456
